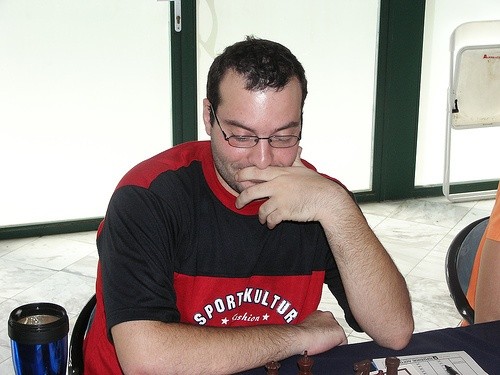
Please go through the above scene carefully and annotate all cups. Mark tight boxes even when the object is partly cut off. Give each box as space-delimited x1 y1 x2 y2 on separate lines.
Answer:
7 301 70 375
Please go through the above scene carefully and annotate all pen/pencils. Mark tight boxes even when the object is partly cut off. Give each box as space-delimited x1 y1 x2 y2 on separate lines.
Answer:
444 365 460 375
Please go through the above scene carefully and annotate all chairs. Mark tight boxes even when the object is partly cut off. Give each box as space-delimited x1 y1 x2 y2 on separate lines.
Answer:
446 216 490 326
442 19 500 203
68 293 98 375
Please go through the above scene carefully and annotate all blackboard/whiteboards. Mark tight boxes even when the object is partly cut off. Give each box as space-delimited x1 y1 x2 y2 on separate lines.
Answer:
449 44 500 131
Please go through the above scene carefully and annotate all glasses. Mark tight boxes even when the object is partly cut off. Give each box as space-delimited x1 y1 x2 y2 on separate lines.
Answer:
210 103 303 148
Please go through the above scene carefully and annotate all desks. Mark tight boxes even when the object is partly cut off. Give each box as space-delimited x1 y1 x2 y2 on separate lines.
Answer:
230 320 500 375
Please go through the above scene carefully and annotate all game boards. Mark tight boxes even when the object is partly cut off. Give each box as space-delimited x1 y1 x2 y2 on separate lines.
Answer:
258 349 401 375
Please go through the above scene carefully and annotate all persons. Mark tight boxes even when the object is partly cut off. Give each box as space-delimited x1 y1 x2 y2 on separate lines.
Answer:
79 36 415 375
456 178 500 325
